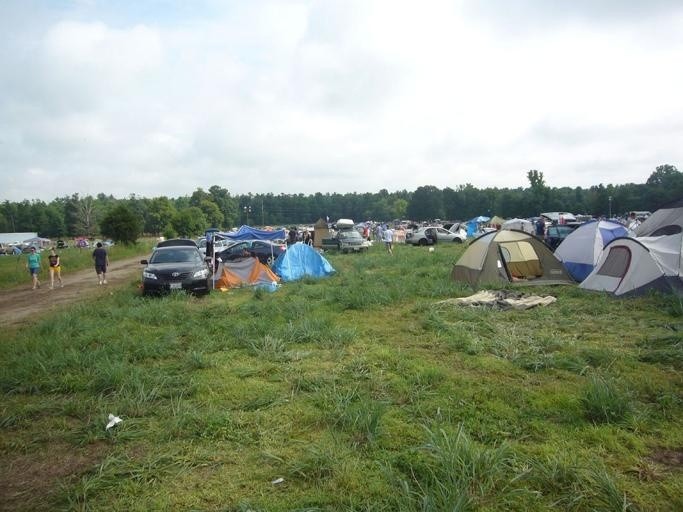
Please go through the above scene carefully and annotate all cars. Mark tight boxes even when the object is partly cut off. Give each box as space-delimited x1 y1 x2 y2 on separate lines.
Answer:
332 230 368 252
546 224 584 248
152 238 197 254
405 225 466 248
196 235 287 267
141 246 210 297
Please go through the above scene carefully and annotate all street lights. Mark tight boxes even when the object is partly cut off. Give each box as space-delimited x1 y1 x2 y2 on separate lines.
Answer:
608 196 612 219
244 205 251 226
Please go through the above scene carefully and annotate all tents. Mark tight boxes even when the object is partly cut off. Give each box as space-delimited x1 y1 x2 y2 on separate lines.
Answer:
448 205 683 301
206 240 337 290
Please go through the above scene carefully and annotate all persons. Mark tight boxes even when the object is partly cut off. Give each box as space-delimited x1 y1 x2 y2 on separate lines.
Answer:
535 207 641 245
407 218 443 236
288 223 313 248
0 236 110 290
363 219 397 257
